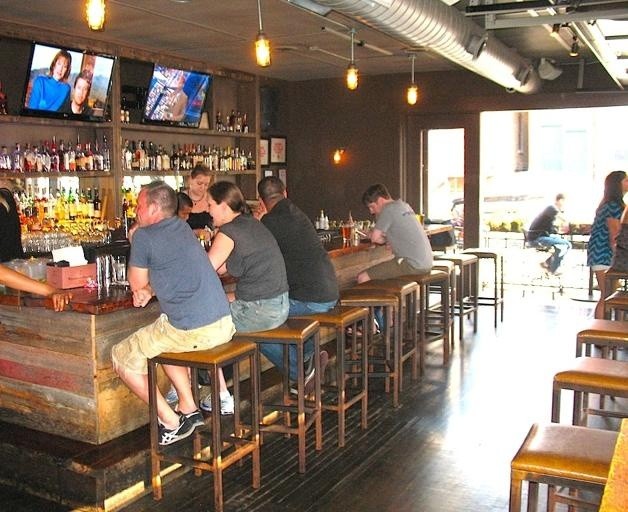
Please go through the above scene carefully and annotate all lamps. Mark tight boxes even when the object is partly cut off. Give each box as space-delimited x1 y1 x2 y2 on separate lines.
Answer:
346 28 358 91
405 55 418 104
254 0 272 67
552 24 581 57
86 0 106 32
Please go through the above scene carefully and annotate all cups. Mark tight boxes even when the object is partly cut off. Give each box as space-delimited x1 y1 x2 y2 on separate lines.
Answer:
341 225 354 241
111 256 126 287
21 231 75 252
96 254 110 286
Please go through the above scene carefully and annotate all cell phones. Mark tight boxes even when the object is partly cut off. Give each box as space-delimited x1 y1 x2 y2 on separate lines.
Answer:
355 229 367 239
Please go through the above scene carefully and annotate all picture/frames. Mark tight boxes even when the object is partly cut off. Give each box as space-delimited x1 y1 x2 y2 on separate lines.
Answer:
260 139 272 168
278 168 287 200
263 169 275 179
270 136 288 166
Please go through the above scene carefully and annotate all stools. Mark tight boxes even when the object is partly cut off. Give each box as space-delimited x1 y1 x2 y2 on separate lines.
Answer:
511 259 628 512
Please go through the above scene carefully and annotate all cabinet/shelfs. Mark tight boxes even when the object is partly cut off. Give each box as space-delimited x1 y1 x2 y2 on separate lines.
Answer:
118 47 259 222
2 25 120 240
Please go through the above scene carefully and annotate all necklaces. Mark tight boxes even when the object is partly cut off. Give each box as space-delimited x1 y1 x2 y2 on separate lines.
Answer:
186 190 204 206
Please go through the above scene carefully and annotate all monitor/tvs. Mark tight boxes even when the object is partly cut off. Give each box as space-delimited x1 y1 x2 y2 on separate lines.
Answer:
140 62 214 131
17 38 119 123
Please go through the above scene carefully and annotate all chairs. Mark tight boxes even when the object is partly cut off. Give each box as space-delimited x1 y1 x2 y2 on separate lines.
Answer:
521 228 567 295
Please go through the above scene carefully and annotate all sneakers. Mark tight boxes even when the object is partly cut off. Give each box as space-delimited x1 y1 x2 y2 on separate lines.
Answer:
199 393 235 416
174 406 206 428
303 350 329 395
290 374 299 395
163 385 180 405
155 415 196 447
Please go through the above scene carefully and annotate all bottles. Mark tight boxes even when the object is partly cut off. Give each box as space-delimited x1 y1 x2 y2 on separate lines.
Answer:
200 234 207 251
319 209 324 230
122 139 256 171
0 134 111 172
17 184 102 219
120 97 130 123
216 109 249 133
324 215 330 230
0 82 8 114
415 214 425 227
314 215 320 232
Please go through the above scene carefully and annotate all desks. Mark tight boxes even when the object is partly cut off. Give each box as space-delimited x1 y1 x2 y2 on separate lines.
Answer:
558 233 602 303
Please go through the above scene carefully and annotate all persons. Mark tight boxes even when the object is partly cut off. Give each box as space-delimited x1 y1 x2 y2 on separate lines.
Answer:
58 68 95 116
127 193 193 246
161 75 188 121
110 181 237 447
0 263 74 313
0 178 23 262
587 171 628 350
527 193 571 276
347 183 433 336
165 180 290 413
27 49 73 112
612 208 628 272
257 176 339 396
184 167 215 229
